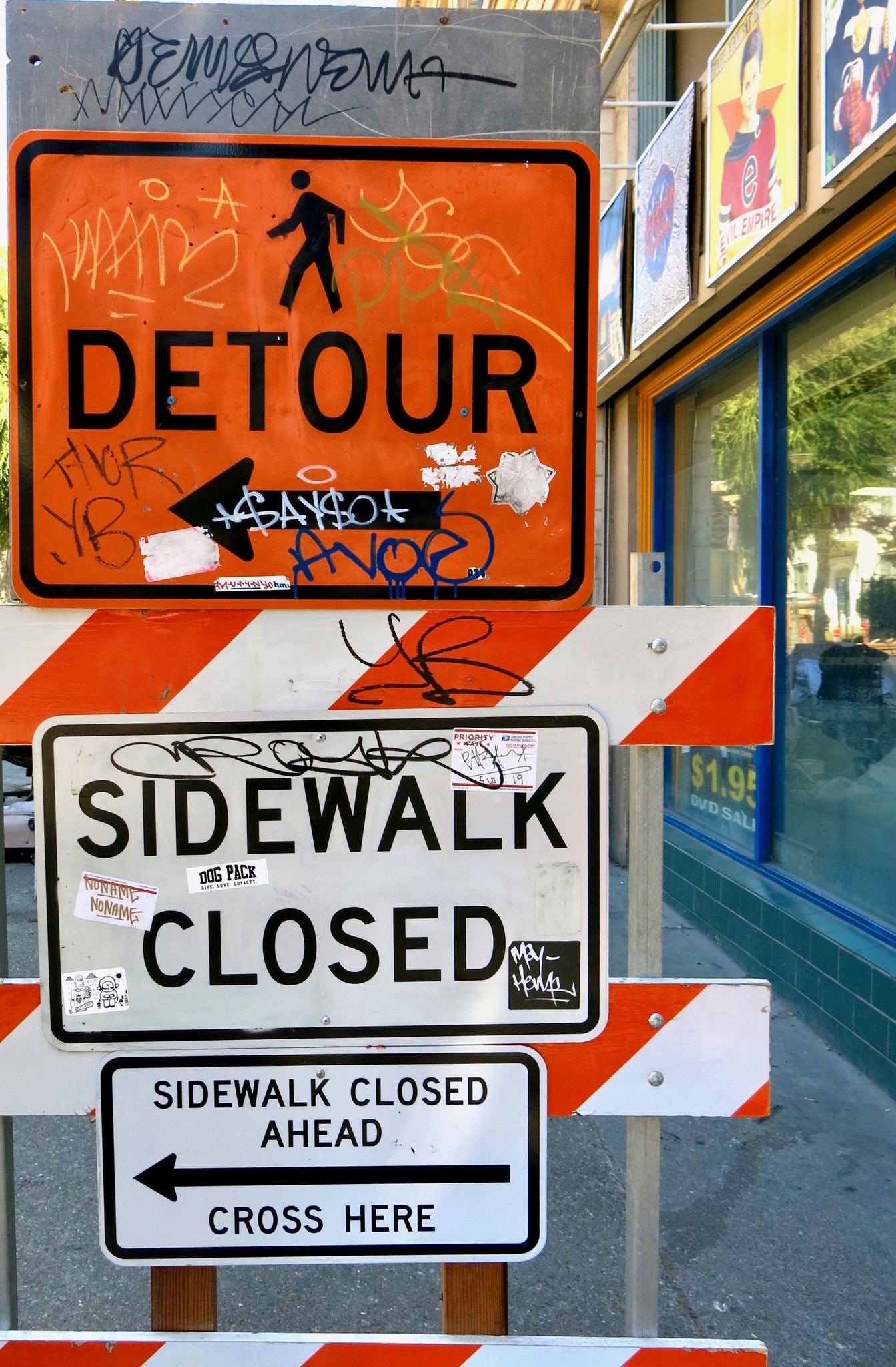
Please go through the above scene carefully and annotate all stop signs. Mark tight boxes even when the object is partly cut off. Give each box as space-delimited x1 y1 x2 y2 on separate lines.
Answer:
97 1054 550 1268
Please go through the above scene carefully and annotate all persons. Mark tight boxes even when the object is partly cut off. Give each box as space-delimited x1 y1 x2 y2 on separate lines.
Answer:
792 626 896 767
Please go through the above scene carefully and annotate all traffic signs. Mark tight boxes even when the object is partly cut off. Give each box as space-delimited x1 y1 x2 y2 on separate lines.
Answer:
10 124 600 609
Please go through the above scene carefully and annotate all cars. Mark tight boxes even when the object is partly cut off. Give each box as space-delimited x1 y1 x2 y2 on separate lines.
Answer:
3 759 37 850
